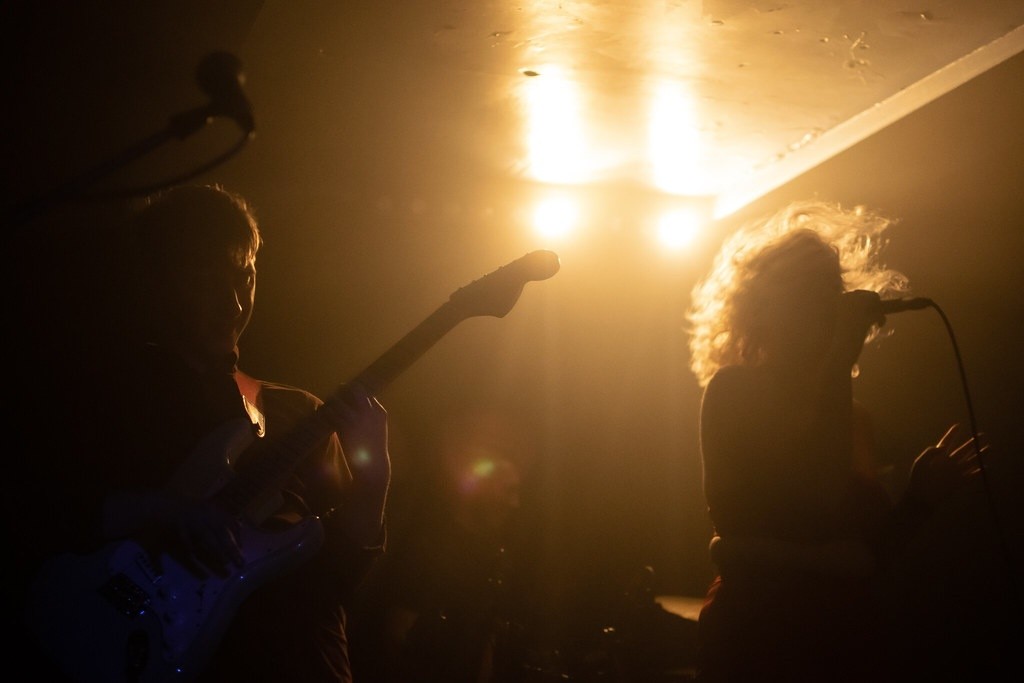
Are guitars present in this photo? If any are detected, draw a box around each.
[228,248,561,503]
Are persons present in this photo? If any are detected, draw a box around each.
[688,186,993,682]
[111,184,392,683]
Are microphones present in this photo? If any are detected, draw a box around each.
[880,295,934,314]
[197,50,257,133]
